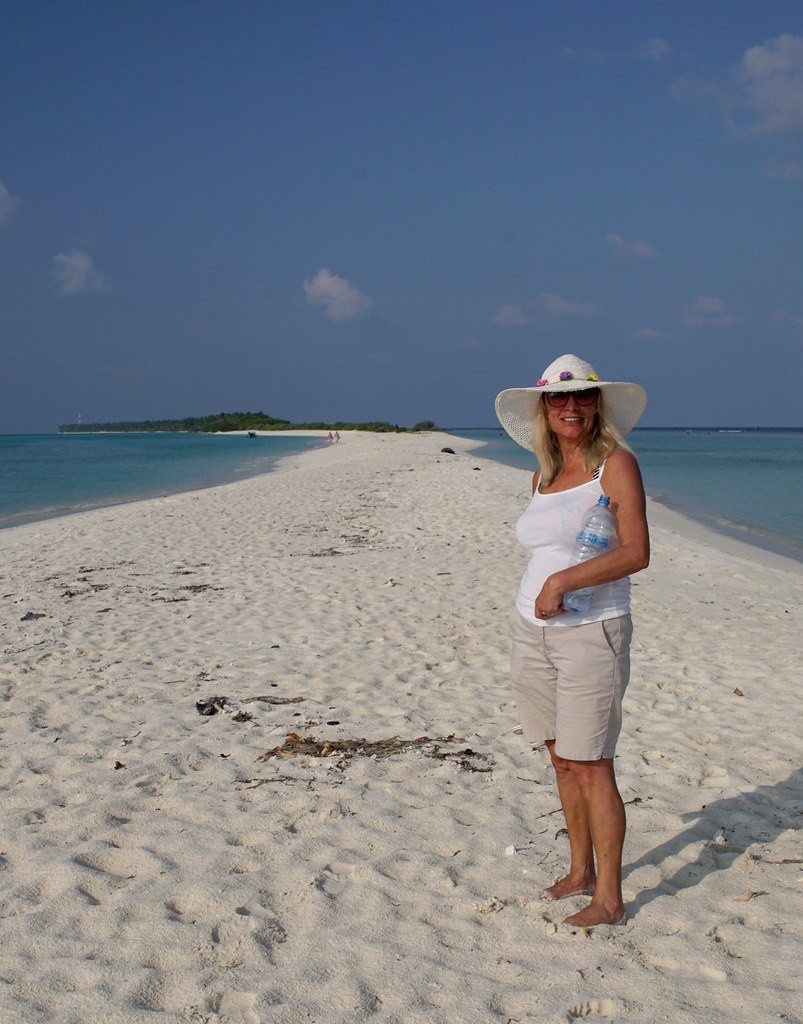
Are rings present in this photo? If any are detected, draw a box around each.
[551,613,554,616]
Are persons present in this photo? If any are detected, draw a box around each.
[494,354,651,928]
[328,431,333,444]
[335,431,339,443]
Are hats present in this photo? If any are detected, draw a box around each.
[495,354,648,453]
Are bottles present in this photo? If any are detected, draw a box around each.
[560,495,614,613]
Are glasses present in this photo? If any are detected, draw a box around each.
[546,388,595,407]
